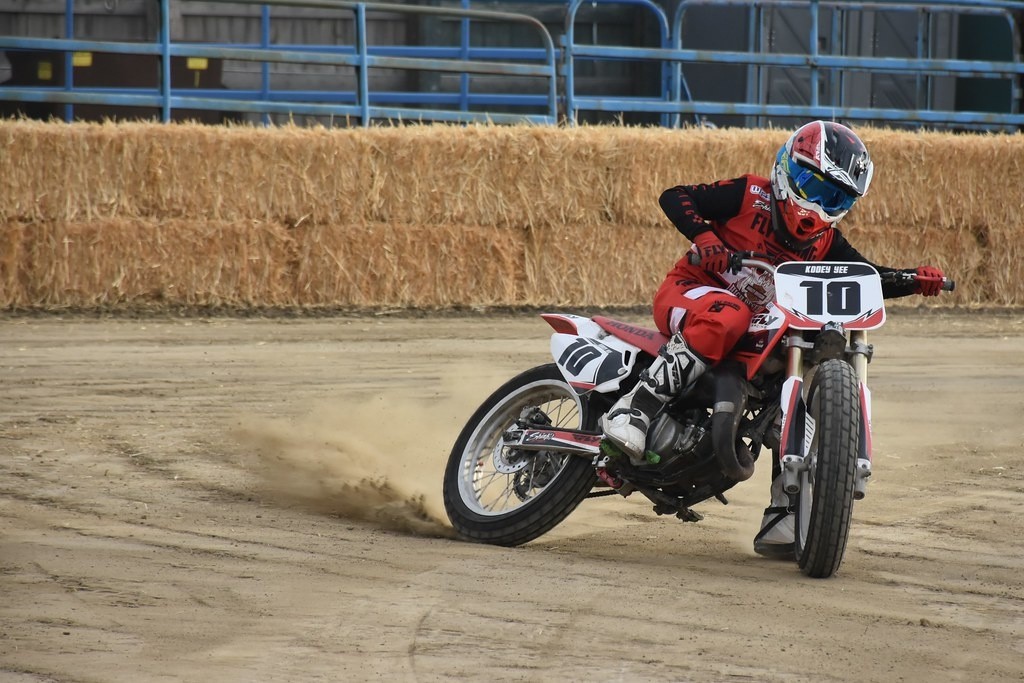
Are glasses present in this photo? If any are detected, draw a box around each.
[794,168,847,211]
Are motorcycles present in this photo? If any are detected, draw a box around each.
[442,247,955,579]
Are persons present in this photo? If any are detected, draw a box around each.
[598,120,944,560]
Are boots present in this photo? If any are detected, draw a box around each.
[754,424,798,561]
[598,331,714,462]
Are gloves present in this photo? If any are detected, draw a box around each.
[896,265,944,297]
[693,230,732,274]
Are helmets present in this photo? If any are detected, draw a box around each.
[770,121,874,252]
